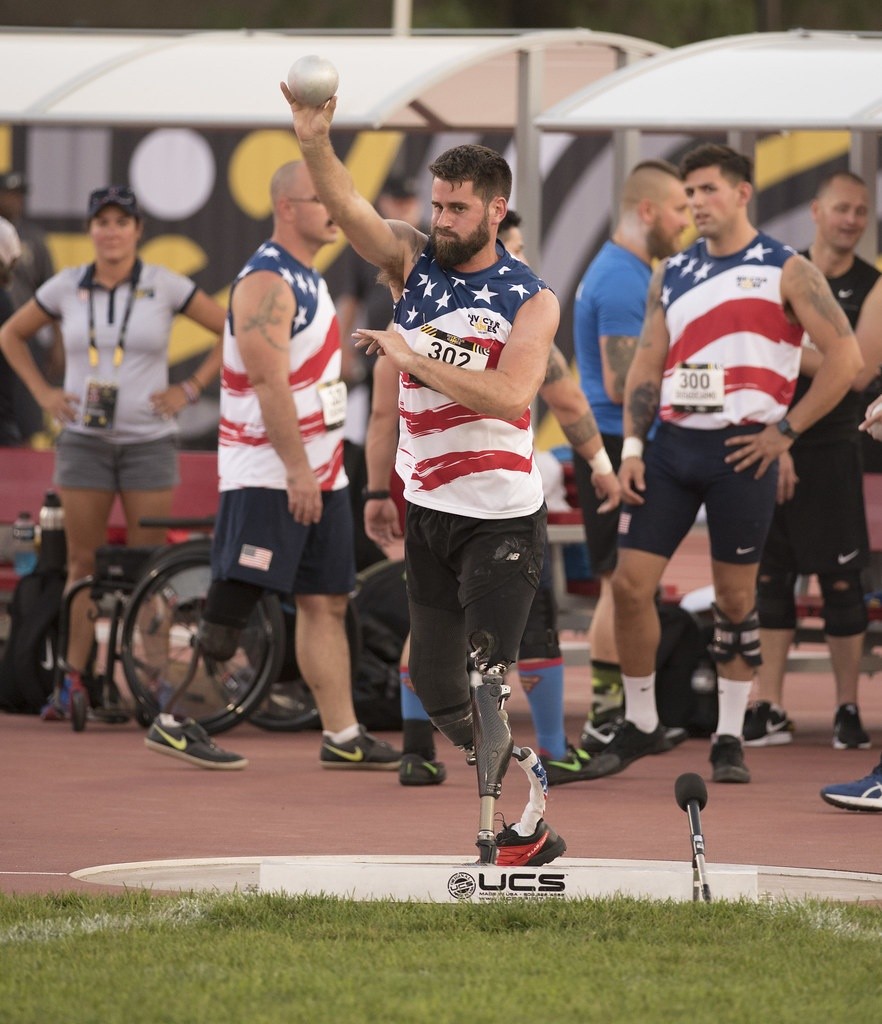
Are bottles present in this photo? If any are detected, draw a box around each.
[38,493,66,566]
[665,581,677,595]
[12,511,36,575]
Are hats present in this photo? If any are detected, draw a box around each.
[88,191,137,219]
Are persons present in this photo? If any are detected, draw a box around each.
[144,158,404,771]
[281,61,569,867]
[1,188,228,725]
[0,158,882,809]
[593,143,867,783]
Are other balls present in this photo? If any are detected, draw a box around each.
[287,54,340,107]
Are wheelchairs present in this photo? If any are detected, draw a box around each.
[53,514,322,734]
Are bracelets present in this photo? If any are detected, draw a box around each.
[187,373,205,396]
[359,486,390,499]
[620,437,644,460]
[588,447,614,475]
[178,380,199,405]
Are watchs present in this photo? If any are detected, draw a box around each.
[776,418,799,440]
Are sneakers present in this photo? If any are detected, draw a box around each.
[831,704,871,750]
[709,735,750,783]
[581,720,671,777]
[542,745,620,784]
[400,752,446,785]
[42,681,90,721]
[319,724,403,770]
[580,707,688,751]
[820,763,882,812]
[144,716,248,770]
[150,684,187,719]
[742,703,793,746]
[476,812,567,866]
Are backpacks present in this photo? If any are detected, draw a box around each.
[0,575,104,714]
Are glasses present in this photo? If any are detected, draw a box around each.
[91,187,133,205]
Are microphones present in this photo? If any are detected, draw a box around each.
[674,772,712,905]
